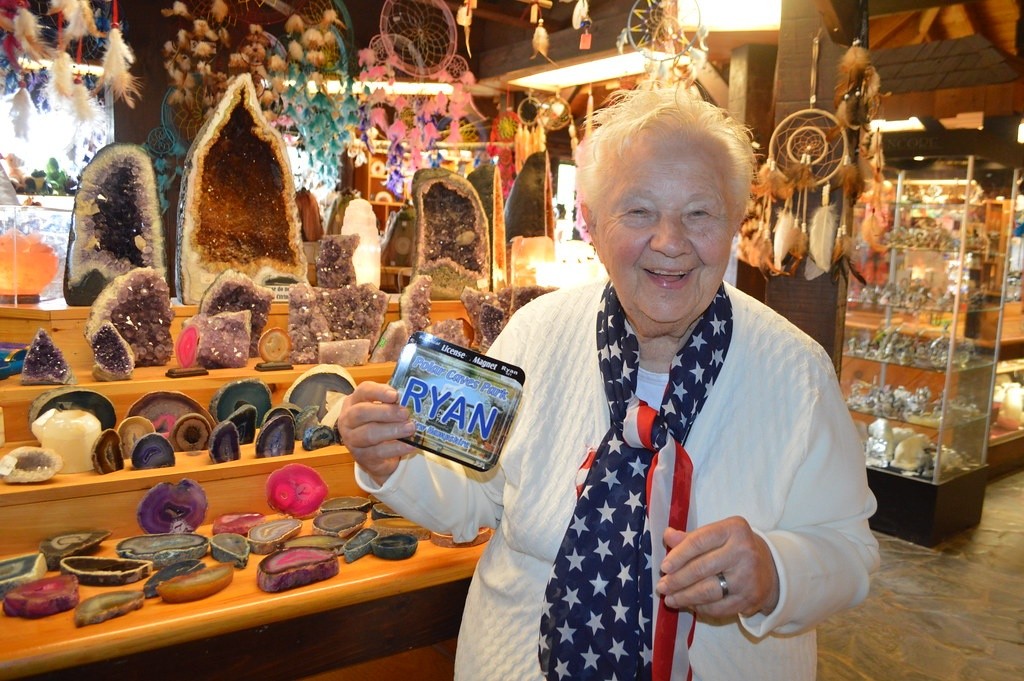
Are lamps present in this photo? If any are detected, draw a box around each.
[479,42,692,96]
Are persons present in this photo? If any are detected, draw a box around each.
[336,88,878,681]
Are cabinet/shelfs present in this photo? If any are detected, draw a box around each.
[844,128,1024,548]
[0,298,488,681]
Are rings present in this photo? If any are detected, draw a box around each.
[717,573,728,598]
